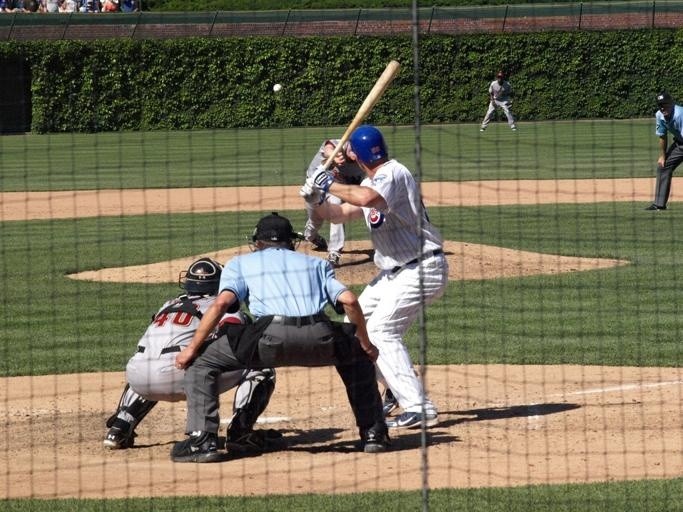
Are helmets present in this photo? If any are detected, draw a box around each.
[349,124,389,164]
[179,256,224,297]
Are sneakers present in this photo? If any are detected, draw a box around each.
[512,127,516,130]
[327,250,340,266]
[103,427,134,448]
[480,128,485,132]
[170,428,218,463]
[383,388,398,415]
[386,410,439,430]
[359,424,391,453]
[226,428,286,452]
[312,235,328,249]
[641,203,666,211]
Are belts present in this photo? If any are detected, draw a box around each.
[137,345,180,353]
[405,247,442,265]
[271,310,324,325]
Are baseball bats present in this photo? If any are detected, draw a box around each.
[322,59,400,167]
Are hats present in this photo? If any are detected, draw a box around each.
[252,212,305,242]
[654,93,672,106]
[496,71,504,78]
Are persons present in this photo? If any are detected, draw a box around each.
[102,257,281,450]
[480,73,518,132]
[171,213,393,463]
[299,125,449,430]
[300,139,351,266]
[1,0,140,13]
[644,93,682,211]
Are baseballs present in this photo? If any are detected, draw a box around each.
[272,84,283,93]
